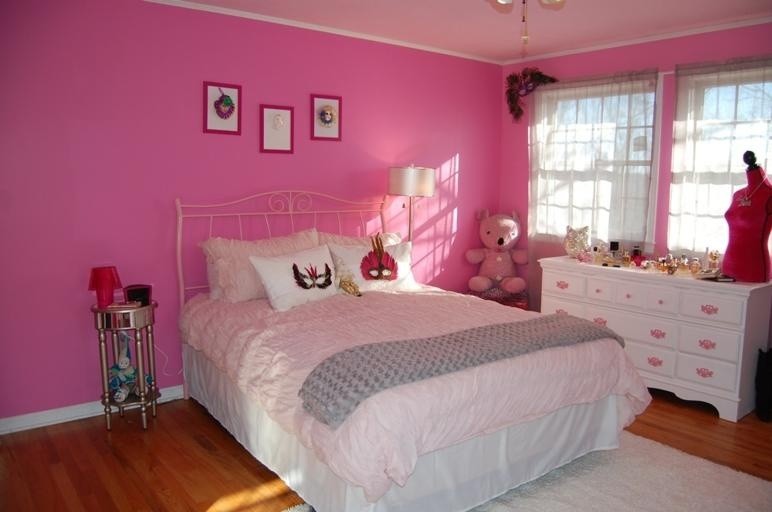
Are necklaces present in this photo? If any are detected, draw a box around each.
[737,175,769,209]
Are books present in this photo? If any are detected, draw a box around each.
[697,267,734,283]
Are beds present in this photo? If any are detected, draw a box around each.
[174,189,653,511]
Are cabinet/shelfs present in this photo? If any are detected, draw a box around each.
[536,256,772,424]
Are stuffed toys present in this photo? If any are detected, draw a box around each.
[463,206,529,296]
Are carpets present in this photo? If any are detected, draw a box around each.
[275,430,772,512]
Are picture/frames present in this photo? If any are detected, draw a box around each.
[202,80,242,136]
[310,94,343,142]
[259,103,295,154]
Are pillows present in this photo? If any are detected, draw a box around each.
[328,241,421,292]
[317,231,402,272]
[197,228,318,304]
[248,244,336,311]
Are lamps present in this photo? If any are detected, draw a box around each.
[487,0,566,59]
[388,163,434,240]
